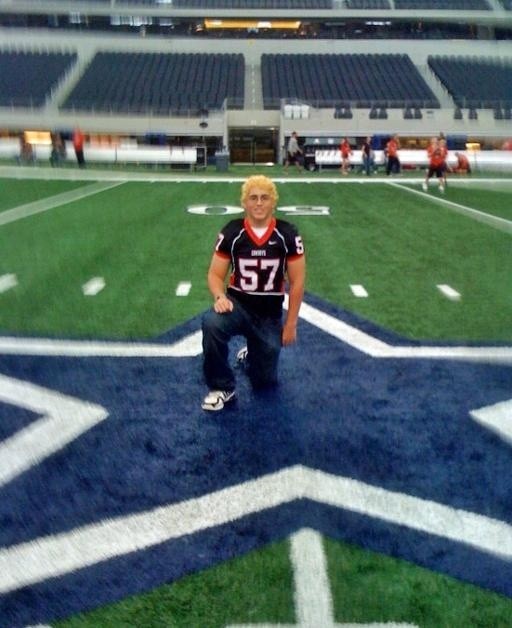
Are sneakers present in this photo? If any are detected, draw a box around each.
[201,390,235,413]
[236,345,249,367]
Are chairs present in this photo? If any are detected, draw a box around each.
[1,50,512,122]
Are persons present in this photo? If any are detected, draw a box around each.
[281,132,471,194]
[201,176,306,411]
[73,126,86,169]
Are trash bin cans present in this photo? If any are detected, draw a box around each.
[215,151,230,171]
